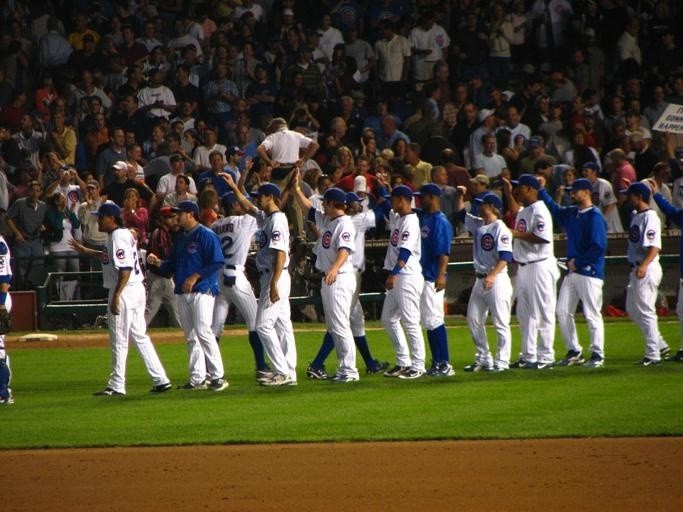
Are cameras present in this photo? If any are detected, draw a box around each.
[63,171,69,177]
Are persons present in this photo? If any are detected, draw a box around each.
[148,171,300,391]
[0,238,15,405]
[455,175,610,373]
[1,1,682,330]
[292,165,454,381]
[649,178,683,363]
[67,203,172,396]
[621,182,671,366]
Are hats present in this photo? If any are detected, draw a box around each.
[267,118,287,129]
[112,161,127,170]
[170,155,185,163]
[86,180,99,189]
[478,108,495,121]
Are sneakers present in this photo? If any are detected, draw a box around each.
[0,395,15,405]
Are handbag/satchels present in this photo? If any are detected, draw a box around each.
[271,167,293,180]
